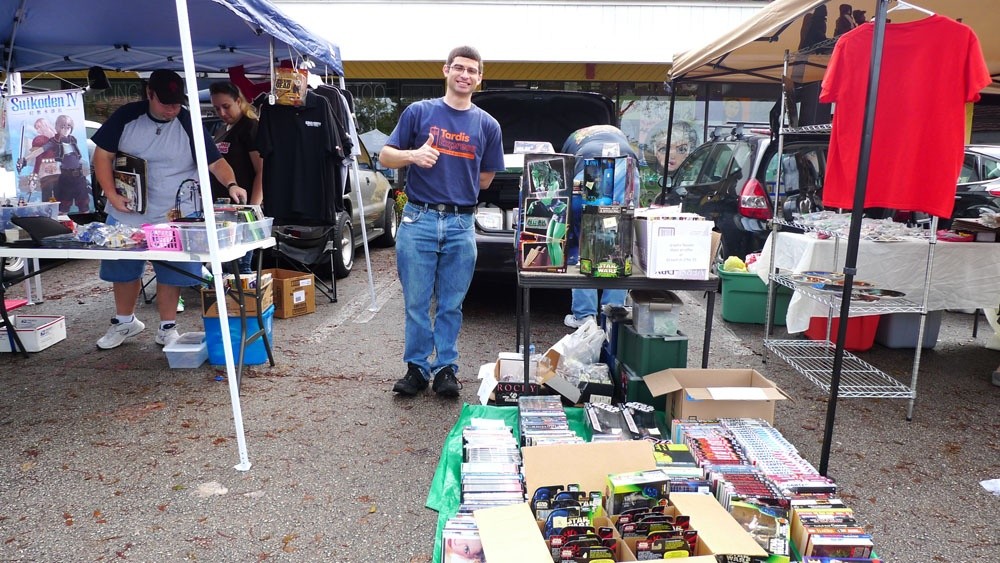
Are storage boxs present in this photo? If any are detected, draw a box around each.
[477,358,614,406]
[476,208,504,230]
[718,264,943,352]
[642,368,796,431]
[253,268,316,319]
[178,223,243,251]
[201,304,274,366]
[200,278,273,317]
[621,324,688,411]
[162,332,208,368]
[629,290,684,335]
[473,440,769,563]
[0,315,66,353]
[0,202,61,233]
[239,217,274,242]
[633,219,714,280]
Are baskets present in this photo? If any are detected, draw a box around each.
[140,222,193,251]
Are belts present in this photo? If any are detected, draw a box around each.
[408,197,477,213]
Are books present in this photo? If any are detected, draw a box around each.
[97,151,148,215]
[173,201,271,289]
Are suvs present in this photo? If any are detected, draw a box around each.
[654,121,911,293]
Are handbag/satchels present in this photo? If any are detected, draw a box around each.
[543,320,606,366]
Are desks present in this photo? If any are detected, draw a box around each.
[756,231,1000,338]
[0,238,277,396]
[516,255,719,395]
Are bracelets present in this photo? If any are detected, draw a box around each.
[227,183,237,190]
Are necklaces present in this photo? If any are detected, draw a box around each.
[150,112,164,135]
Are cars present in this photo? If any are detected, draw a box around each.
[911,144,1000,231]
[470,89,617,296]
[334,133,401,278]
[83,120,102,191]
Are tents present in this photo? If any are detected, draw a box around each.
[0,0,373,467]
[660,0,1000,476]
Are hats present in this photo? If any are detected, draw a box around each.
[149,69,188,106]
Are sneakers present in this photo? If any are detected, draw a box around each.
[155,323,180,345]
[431,366,463,397]
[392,360,429,397]
[177,296,185,311]
[97,314,145,349]
[564,314,591,327]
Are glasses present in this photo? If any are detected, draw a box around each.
[447,65,481,75]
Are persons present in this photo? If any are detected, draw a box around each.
[91,68,245,351]
[205,81,264,279]
[378,47,505,395]
[561,125,640,329]
[445,539,483,560]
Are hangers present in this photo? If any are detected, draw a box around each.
[300,60,324,90]
[871,0,934,21]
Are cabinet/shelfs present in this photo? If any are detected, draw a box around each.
[763,35,938,422]
